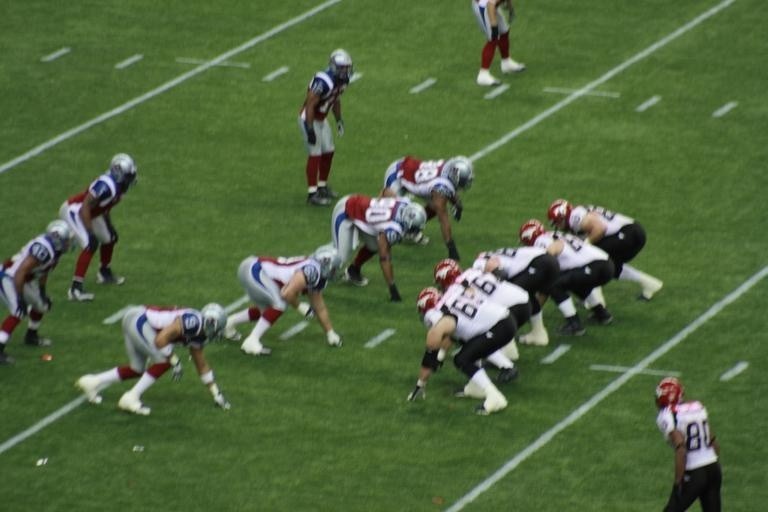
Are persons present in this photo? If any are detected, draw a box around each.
[330,194,428,302]
[471,1,527,88]
[0,219,72,365]
[58,153,137,301]
[406,200,665,416]
[297,48,355,207]
[652,376,724,512]
[74,302,230,416]
[377,154,475,262]
[221,245,343,356]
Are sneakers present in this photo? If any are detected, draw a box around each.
[25,338,51,346]
[241,341,271,357]
[561,303,611,330]
[74,376,150,415]
[638,274,662,297]
[223,326,242,341]
[477,69,499,86]
[0,355,14,365]
[501,59,525,74]
[459,367,517,413]
[405,231,430,246]
[308,187,339,202]
[70,268,126,299]
[344,268,369,287]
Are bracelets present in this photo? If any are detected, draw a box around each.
[199,369,215,386]
[160,343,175,358]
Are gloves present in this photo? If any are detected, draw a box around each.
[212,392,230,411]
[388,284,401,302]
[89,227,118,248]
[450,194,462,221]
[336,120,343,136]
[326,331,343,348]
[16,292,27,317]
[446,240,460,258]
[39,285,51,310]
[408,379,427,402]
[307,126,315,144]
[297,303,315,320]
[170,360,182,384]
[491,25,498,38]
[672,483,683,498]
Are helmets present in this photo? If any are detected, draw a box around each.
[111,154,137,184]
[520,197,572,241]
[449,160,473,189]
[329,49,353,80]
[419,261,461,310]
[200,303,227,344]
[314,247,341,278]
[655,377,683,408]
[46,220,76,253]
[402,203,427,230]
